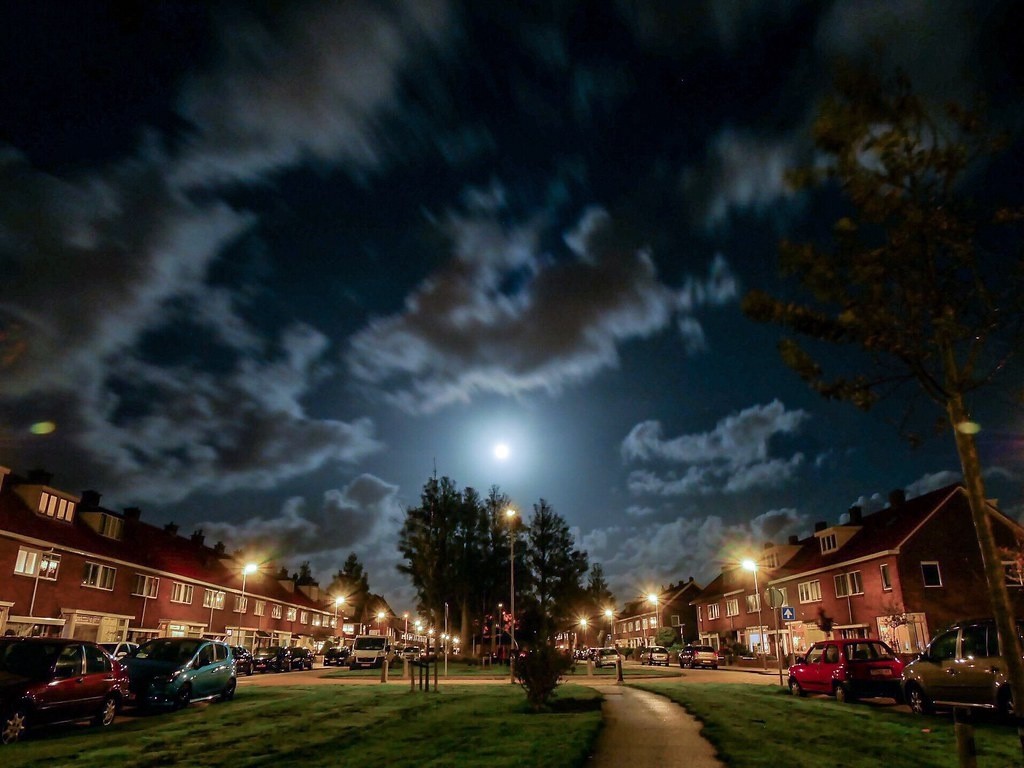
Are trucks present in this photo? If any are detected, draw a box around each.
[353,634,395,669]
[396,641,461,664]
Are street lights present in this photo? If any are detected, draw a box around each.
[403,613,409,646]
[333,595,344,631]
[649,593,660,646]
[377,612,384,629]
[504,507,517,683]
[236,561,258,644]
[580,618,588,645]
[747,562,768,670]
[607,610,614,646]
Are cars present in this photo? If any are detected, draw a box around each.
[593,647,622,668]
[900,618,1019,727]
[571,647,597,661]
[230,645,254,676]
[96,641,147,661]
[288,646,306,672]
[118,637,237,715]
[252,646,292,674]
[640,646,670,668]
[678,644,719,670]
[787,638,907,704]
[303,648,314,670]
[324,646,353,666]
[0,635,130,747]
